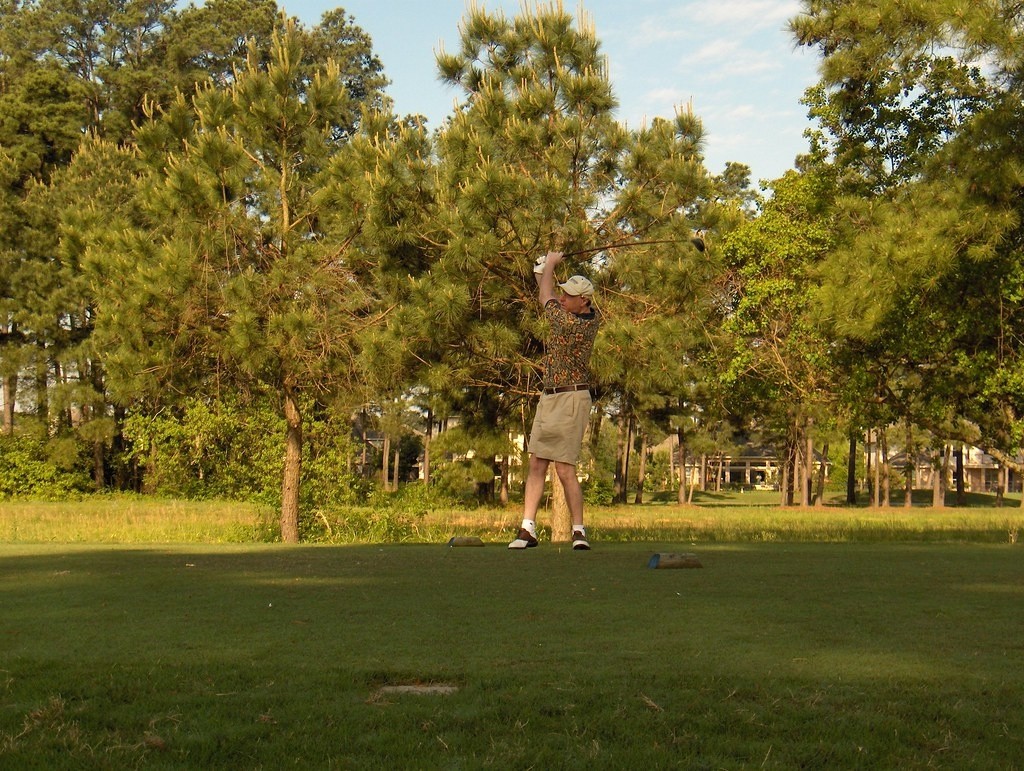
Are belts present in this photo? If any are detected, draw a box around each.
[544,384,589,395]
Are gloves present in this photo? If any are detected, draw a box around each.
[534,256,546,273]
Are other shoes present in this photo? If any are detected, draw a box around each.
[508,528,538,549]
[572,530,590,550]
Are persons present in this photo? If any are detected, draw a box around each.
[508,251,597,548]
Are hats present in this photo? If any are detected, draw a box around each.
[555,275,594,300]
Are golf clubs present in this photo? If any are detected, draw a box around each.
[534,238,706,266]
